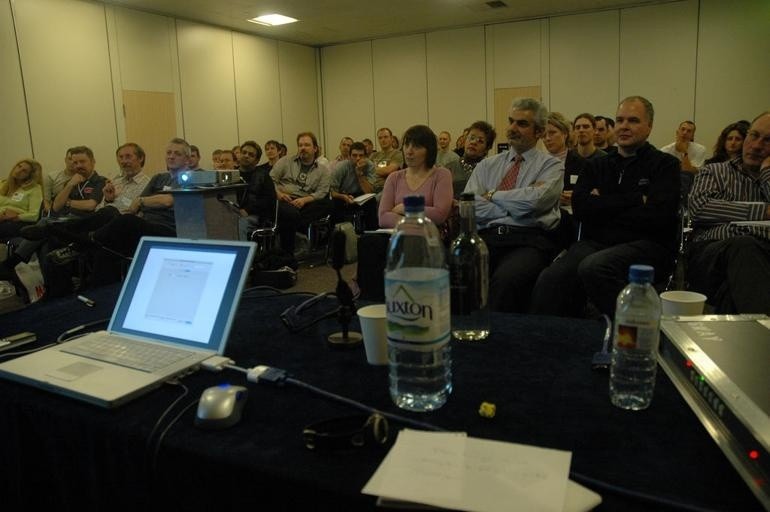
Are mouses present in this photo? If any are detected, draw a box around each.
[194,383,249,426]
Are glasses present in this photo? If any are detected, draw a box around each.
[466,135,486,144]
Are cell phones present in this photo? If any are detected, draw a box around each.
[0,331,37,352]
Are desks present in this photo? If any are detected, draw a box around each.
[0,283,770,512]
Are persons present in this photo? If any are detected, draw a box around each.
[1,112,770,325]
[451,97,567,318]
[529,96,684,317]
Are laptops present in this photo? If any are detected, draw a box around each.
[0,234,257,412]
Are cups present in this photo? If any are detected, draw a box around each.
[658,287,707,315]
[356,304,390,367]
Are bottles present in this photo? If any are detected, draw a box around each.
[454,191,493,344]
[608,265,661,413]
[383,194,454,412]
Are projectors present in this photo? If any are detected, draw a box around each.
[177,169,241,186]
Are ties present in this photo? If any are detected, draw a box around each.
[497,155,524,191]
